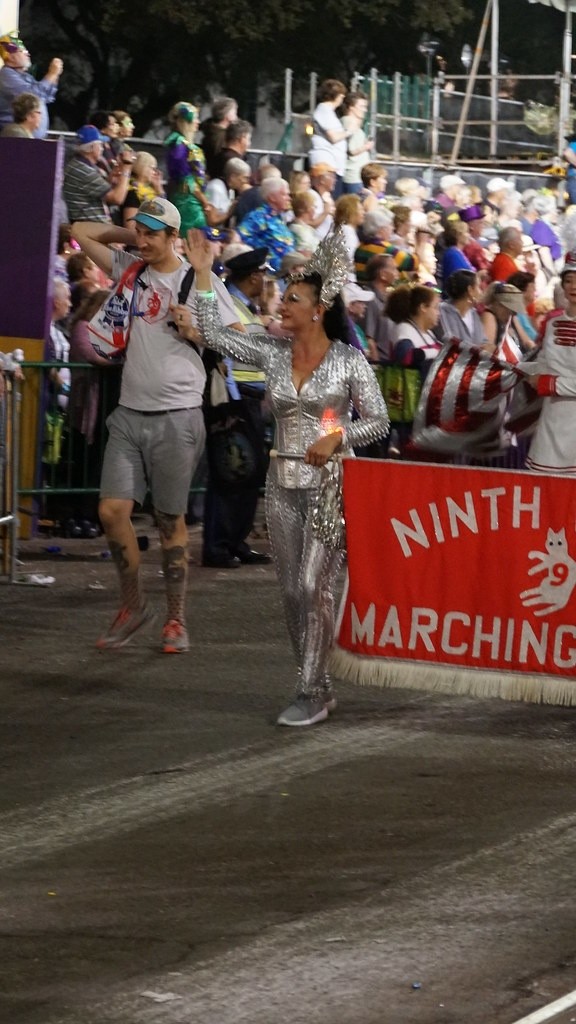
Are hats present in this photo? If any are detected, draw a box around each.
[458,205,486,221]
[223,246,277,276]
[494,283,524,312]
[486,177,513,193]
[77,124,111,144]
[310,162,337,176]
[440,174,466,188]
[560,249,576,274]
[340,283,375,305]
[520,235,540,252]
[362,205,395,234]
[125,197,182,234]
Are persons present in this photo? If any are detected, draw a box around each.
[0,30,576,571]
[72,196,246,652]
[184,228,390,728]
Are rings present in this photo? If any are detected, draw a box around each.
[180,315,182,320]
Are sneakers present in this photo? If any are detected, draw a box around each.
[323,693,335,711]
[98,600,153,649]
[161,617,188,653]
[275,690,329,726]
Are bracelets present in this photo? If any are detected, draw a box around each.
[197,290,216,297]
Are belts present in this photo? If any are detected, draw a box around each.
[128,407,193,417]
[234,384,265,401]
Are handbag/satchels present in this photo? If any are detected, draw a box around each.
[373,363,420,423]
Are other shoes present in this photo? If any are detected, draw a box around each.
[238,551,273,566]
[201,549,239,568]
